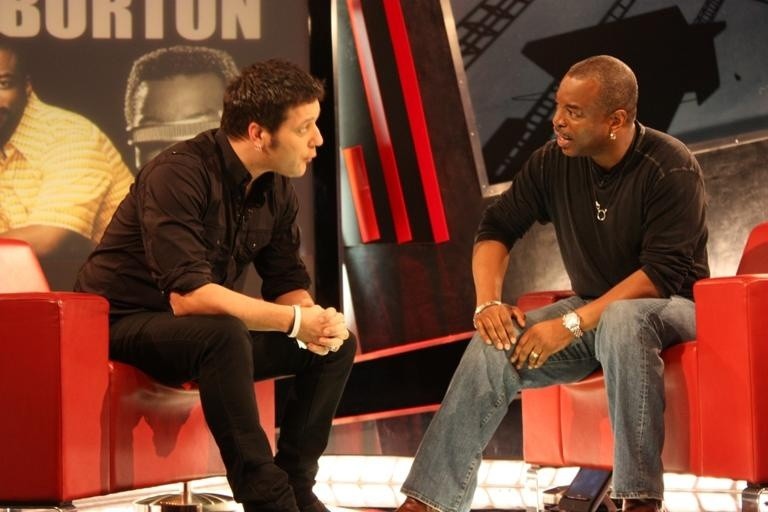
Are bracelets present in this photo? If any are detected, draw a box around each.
[288,304,302,338]
[472,300,502,330]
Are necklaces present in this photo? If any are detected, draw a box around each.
[586,145,627,223]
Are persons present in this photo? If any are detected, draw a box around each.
[392,51,715,511]
[80,56,359,512]
[122,45,247,171]
[0,31,139,298]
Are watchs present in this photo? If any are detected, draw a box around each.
[562,311,585,337]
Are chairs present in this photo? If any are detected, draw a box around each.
[514,220,768,511]
[0,234,277,509]
